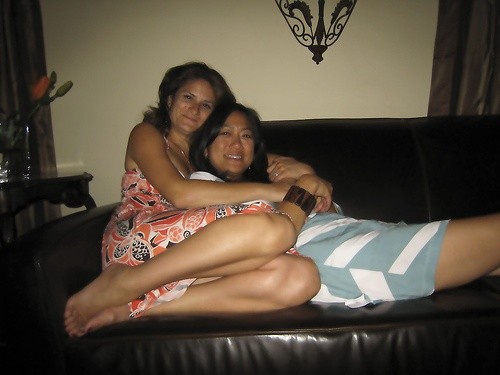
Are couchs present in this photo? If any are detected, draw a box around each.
[13,114,500,375]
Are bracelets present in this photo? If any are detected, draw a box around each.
[283,184,316,218]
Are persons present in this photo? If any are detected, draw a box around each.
[63,61,321,341]
[190,102,500,308]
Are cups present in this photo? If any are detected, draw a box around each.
[0,153,14,184]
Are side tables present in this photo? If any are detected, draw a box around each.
[0,171,96,245]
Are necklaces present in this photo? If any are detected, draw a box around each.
[174,144,185,157]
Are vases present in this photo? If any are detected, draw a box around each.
[3,113,38,176]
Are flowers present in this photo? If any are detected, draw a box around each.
[0,71,73,152]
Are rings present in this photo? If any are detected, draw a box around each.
[275,171,279,177]
